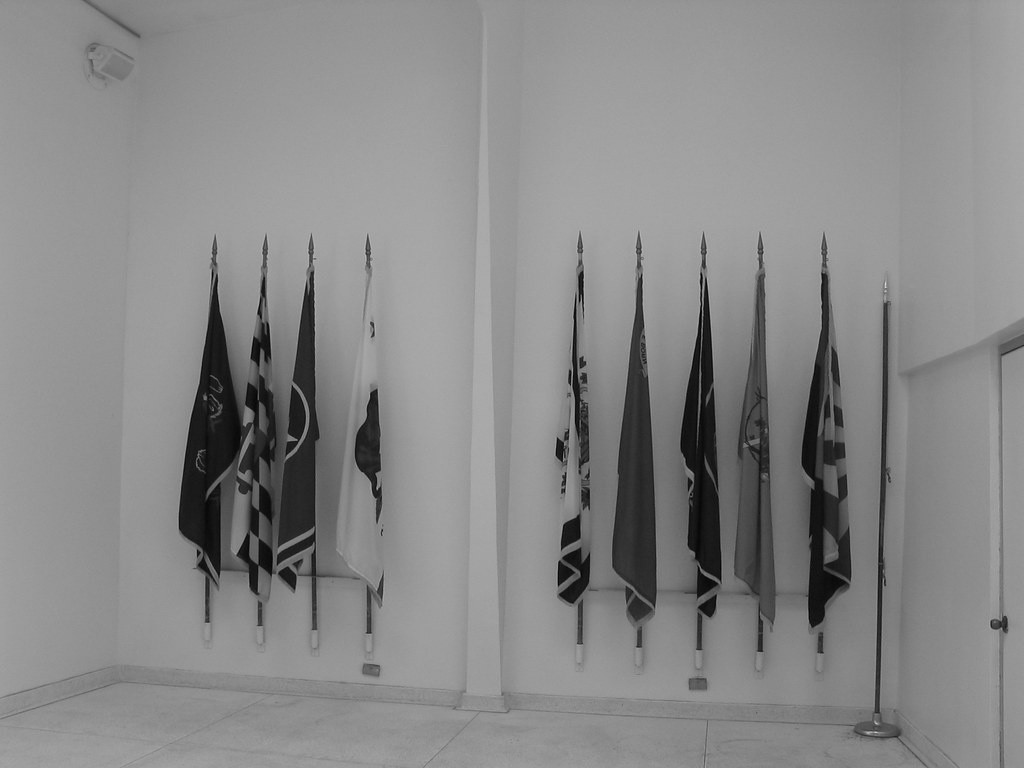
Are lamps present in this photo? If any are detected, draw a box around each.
[84,42,134,86]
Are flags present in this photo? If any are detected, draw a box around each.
[177,271,240,591]
[735,271,776,633]
[801,295,853,649]
[231,274,275,603]
[678,269,723,619]
[611,279,658,629]
[277,269,319,593]
[553,261,592,607]
[333,275,384,609]
